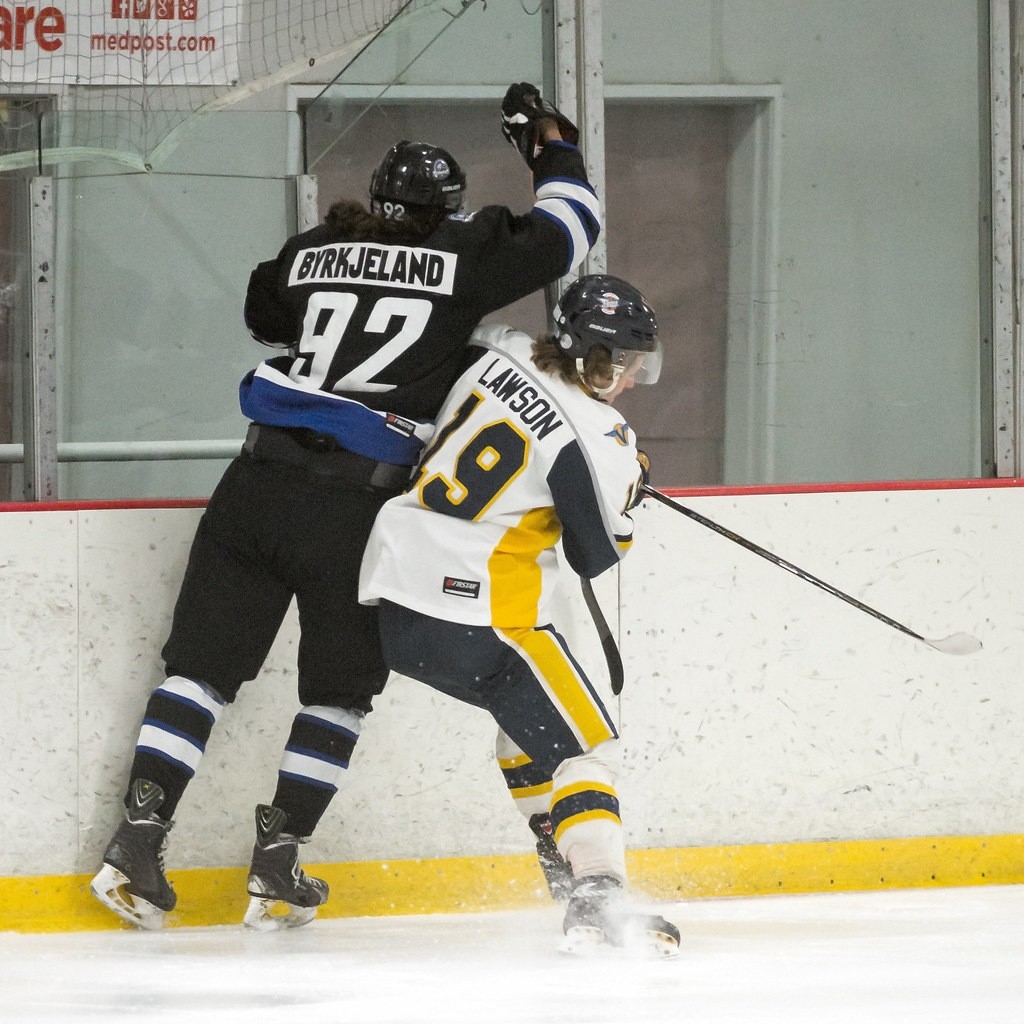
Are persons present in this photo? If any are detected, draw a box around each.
[354,272,685,961]
[93,81,603,928]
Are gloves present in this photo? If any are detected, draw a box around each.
[500,82,580,172]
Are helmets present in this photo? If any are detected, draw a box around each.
[551,274,660,394]
[368,140,467,213]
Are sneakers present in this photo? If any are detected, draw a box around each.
[558,876,680,965]
[89,778,177,930]
[242,804,329,933]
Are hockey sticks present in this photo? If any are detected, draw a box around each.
[542,283,984,696]
